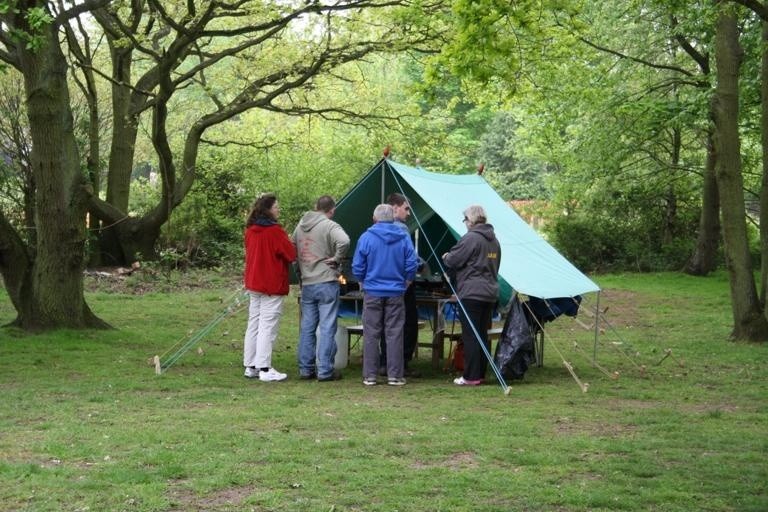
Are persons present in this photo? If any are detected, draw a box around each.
[442,206,501,386]
[380,192,421,377]
[290,196,350,381]
[352,204,418,385]
[244,195,296,382]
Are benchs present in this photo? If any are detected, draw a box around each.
[483,327,505,357]
[346,321,427,366]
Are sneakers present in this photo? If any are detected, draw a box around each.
[243,366,260,378]
[362,377,377,385]
[300,371,318,381]
[403,368,424,379]
[387,377,408,386]
[378,367,387,376]
[319,370,343,381]
[453,376,487,387]
[259,367,288,382]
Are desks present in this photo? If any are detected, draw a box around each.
[297,294,459,370]
